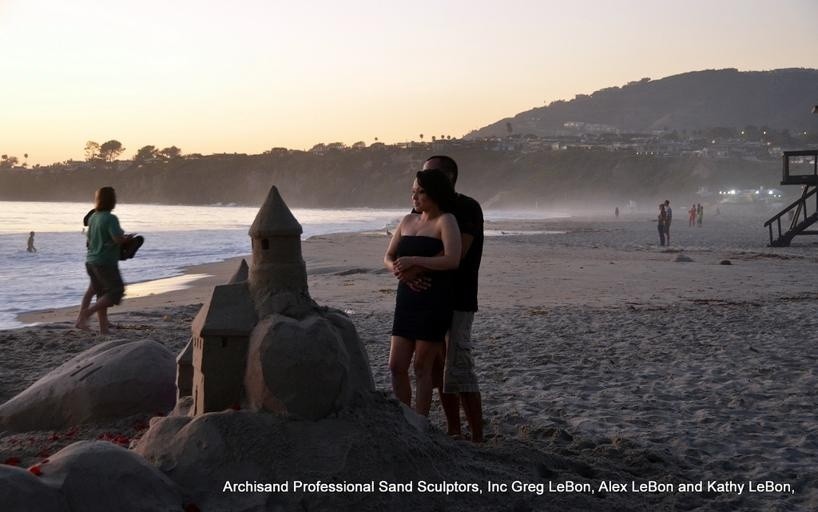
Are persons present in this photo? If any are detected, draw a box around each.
[25,231,37,253]
[651,204,667,246]
[77,187,137,337]
[696,203,701,224]
[662,200,673,245]
[688,205,697,227]
[393,155,484,443]
[74,208,118,331]
[383,168,463,418]
[699,206,704,225]
